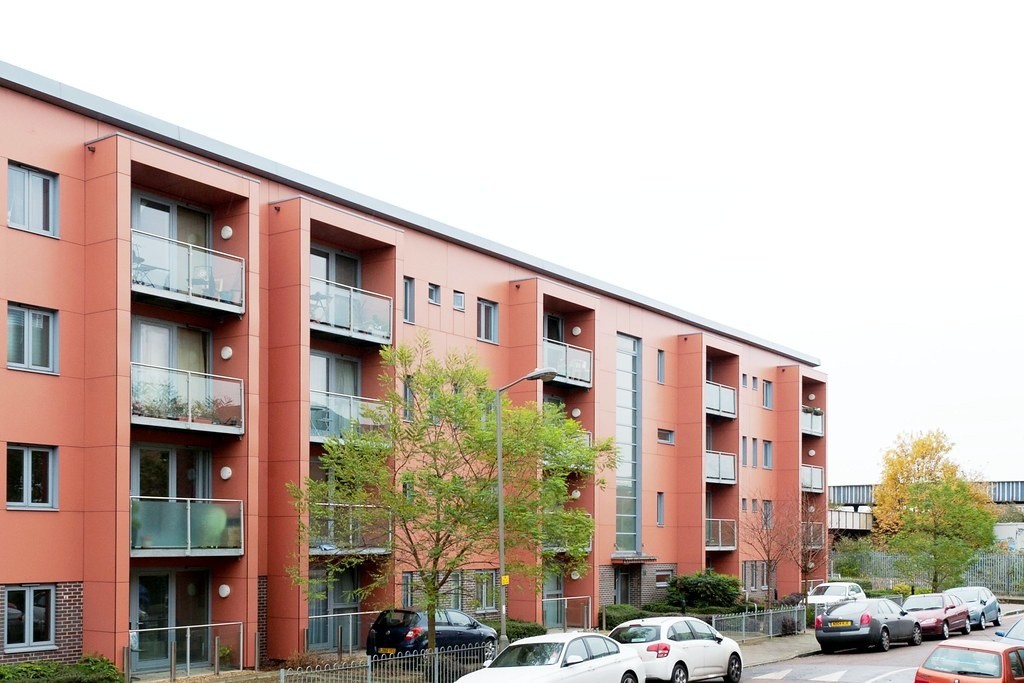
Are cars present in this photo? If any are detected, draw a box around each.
[945,585,1001,629]
[915,636,1024,683]
[606,615,742,683]
[453,632,646,683]
[797,582,865,610]
[365,605,498,661]
[993,617,1024,643]
[815,598,922,652]
[901,592,971,639]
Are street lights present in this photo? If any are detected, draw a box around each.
[493,366,559,659]
